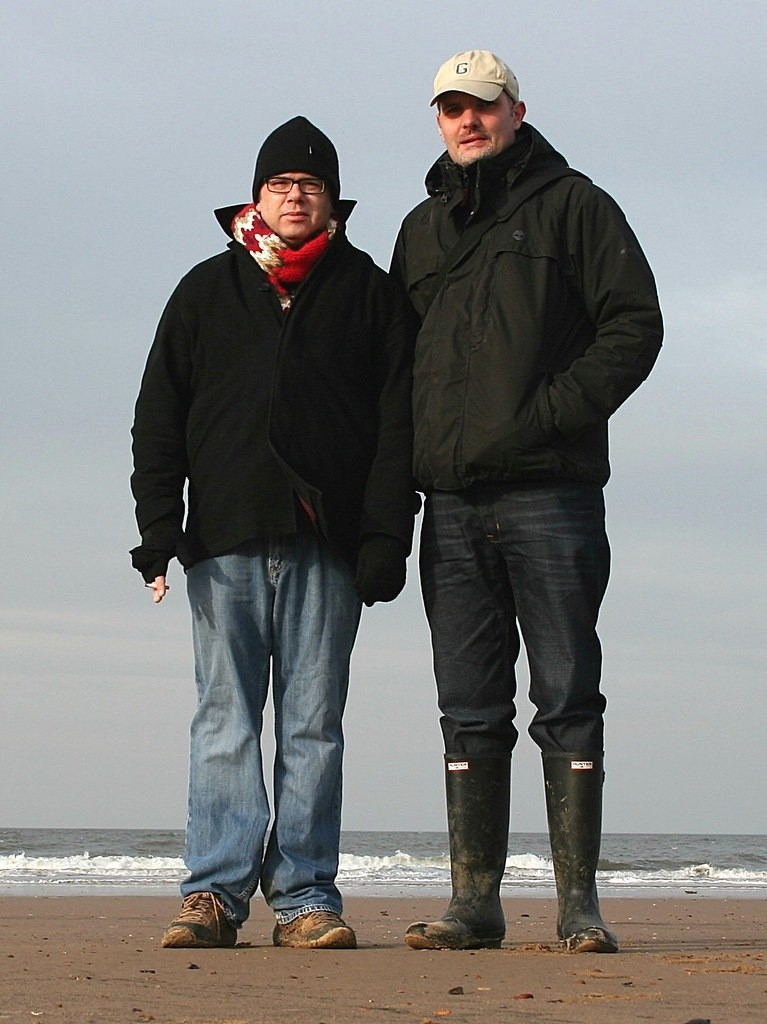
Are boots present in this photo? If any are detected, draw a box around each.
[541,749,617,952]
[404,751,512,950]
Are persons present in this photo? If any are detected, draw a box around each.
[129,114,423,948]
[388,47,665,954]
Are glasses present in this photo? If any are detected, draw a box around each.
[262,175,328,194]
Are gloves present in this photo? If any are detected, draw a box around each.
[353,533,408,607]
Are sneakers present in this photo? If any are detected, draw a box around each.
[273,905,357,949]
[161,883,237,950]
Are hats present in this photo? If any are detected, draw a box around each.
[252,116,341,204]
[429,49,519,107]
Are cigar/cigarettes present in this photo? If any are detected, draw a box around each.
[144,583,169,590]
